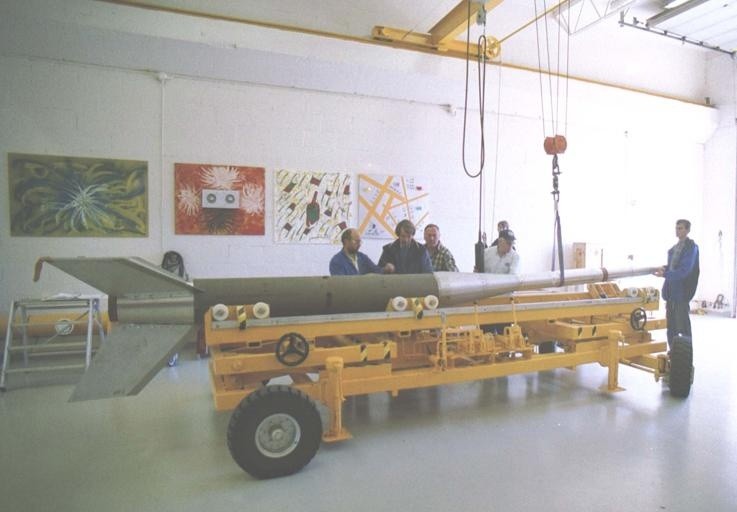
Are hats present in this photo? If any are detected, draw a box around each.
[499,230,514,240]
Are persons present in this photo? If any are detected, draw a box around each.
[422,223,458,273]
[330,229,394,276]
[652,220,699,399]
[482,221,515,251]
[472,230,518,275]
[377,219,433,274]
